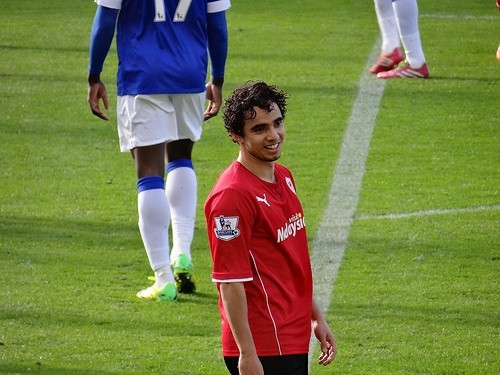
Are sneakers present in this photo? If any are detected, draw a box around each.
[174,255,198,293]
[370,47,404,74]
[136,284,179,302]
[377,64,429,78]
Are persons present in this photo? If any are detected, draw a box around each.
[369,0,430,79]
[86,0,232,301]
[203,78,338,375]
